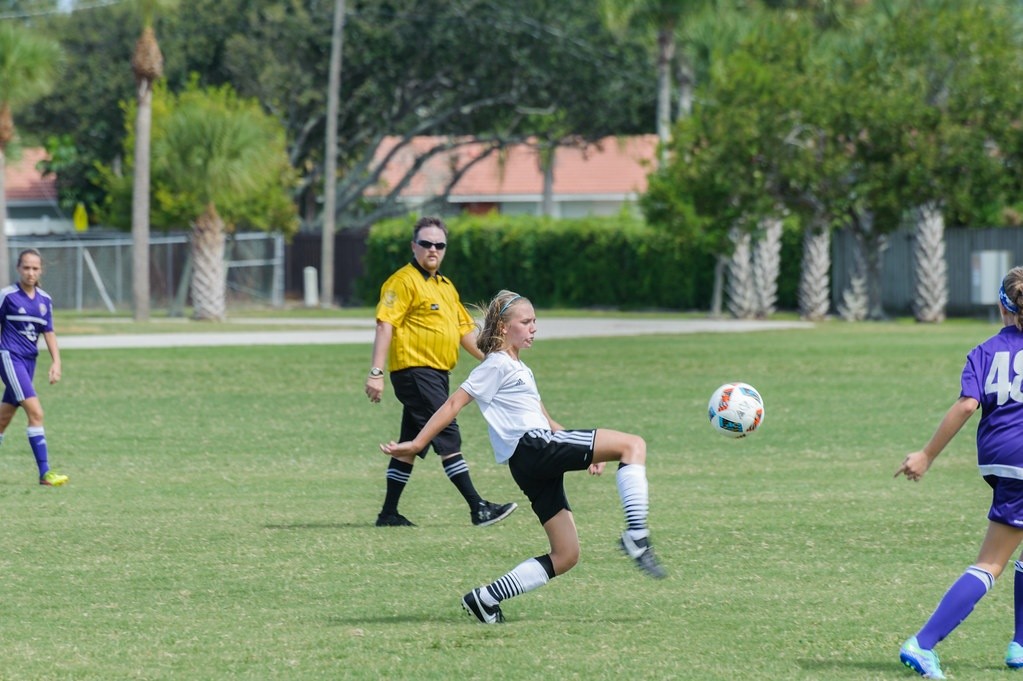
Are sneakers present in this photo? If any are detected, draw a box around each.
[39,470,68,486]
[1005,642,1023,669]
[470,500,518,527]
[898,636,948,679]
[461,588,506,624]
[617,530,667,580]
[376,513,417,527]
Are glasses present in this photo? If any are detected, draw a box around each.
[415,240,447,250]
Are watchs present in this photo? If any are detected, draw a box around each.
[369,367,384,378]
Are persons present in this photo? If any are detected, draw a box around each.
[379,290,672,624]
[893,265,1023,679]
[0,247,69,486]
[365,218,517,526]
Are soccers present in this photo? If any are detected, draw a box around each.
[707,381,765,439]
